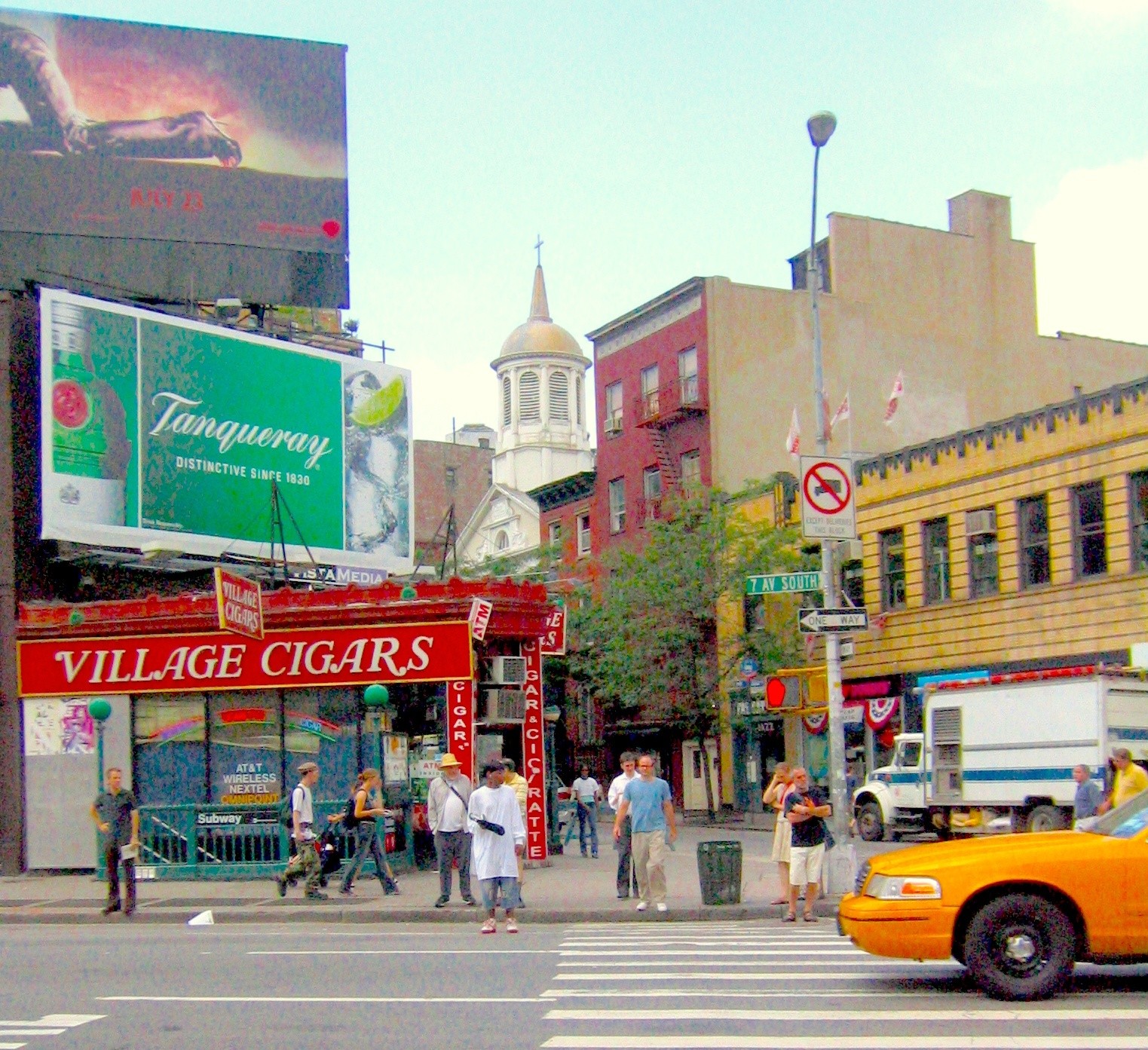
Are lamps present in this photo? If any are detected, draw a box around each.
[215,298,242,320]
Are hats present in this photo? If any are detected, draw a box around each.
[298,762,317,773]
[440,753,462,768]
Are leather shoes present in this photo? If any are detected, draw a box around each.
[617,893,629,899]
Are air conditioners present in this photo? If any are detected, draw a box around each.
[836,539,863,561]
[604,418,622,434]
[481,654,526,686]
[478,689,526,724]
[965,510,998,536]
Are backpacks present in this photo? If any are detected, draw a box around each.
[343,789,367,830]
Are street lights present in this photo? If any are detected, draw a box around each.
[802,107,858,843]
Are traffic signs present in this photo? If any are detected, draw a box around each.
[797,606,869,634]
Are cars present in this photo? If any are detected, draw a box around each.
[832,785,1148,1002]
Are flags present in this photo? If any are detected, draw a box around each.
[786,406,805,463]
[883,369,905,426]
[830,390,850,435]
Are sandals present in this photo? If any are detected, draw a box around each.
[803,911,818,921]
[781,911,795,922]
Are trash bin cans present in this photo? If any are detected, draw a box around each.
[697,841,743,905]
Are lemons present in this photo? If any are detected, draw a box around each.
[348,375,404,426]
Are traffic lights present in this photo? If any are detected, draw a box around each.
[763,674,803,712]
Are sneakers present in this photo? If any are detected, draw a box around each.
[481,918,498,933]
[461,892,476,907]
[636,902,648,912]
[434,895,451,908]
[656,902,667,913]
[505,917,518,933]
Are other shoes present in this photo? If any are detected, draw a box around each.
[123,898,136,915]
[102,900,121,914]
[771,896,789,905]
[382,883,400,895]
[272,873,287,897]
[337,886,357,898]
[304,888,328,901]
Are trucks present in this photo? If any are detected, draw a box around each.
[848,658,1148,843]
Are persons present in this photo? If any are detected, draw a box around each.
[89,768,139,915]
[327,768,403,897]
[608,752,679,913]
[780,765,833,922]
[1096,747,1148,817]
[427,753,476,908]
[762,761,807,906]
[1072,764,1106,831]
[572,765,600,860]
[275,761,329,902]
[468,758,529,933]
[844,763,859,807]
